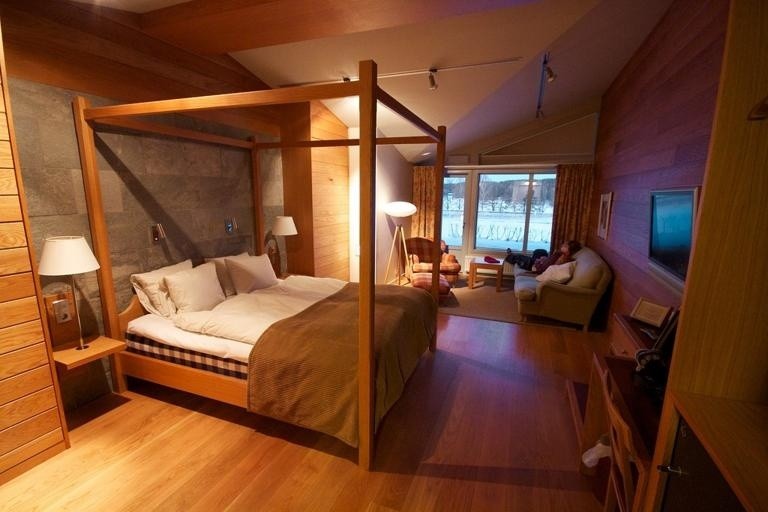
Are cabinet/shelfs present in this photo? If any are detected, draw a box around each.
[51,336,127,371]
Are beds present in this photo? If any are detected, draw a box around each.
[71,58,450,470]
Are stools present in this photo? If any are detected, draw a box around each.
[410,272,451,305]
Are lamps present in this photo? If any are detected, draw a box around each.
[149,223,167,243]
[426,73,439,90]
[272,216,299,276]
[542,59,546,83]
[37,235,100,350]
[383,201,417,286]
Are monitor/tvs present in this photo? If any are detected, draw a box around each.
[646,185,699,293]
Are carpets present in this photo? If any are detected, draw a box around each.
[402,270,577,331]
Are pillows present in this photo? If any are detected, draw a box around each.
[224,253,278,295]
[134,260,194,319]
[535,262,568,281]
[203,252,250,297]
[163,262,225,314]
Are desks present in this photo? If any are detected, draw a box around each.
[469,255,505,292]
[568,309,671,509]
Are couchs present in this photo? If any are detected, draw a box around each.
[408,238,461,287]
[515,248,611,332]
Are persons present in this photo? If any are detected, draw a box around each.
[506,240,581,275]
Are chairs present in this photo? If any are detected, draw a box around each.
[597,372,636,512]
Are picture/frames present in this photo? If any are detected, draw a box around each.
[596,192,613,241]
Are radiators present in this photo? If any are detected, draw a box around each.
[464,255,515,275]
